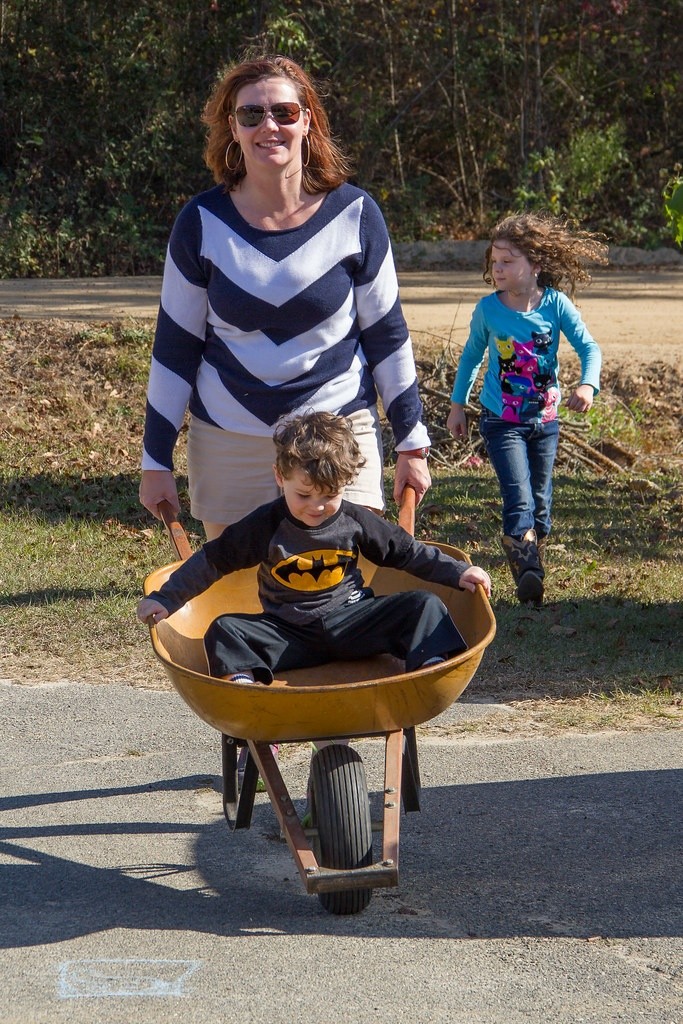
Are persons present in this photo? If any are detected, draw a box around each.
[447,207,610,608]
[137,407,491,685]
[139,55,432,829]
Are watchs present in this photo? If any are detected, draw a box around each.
[397,447,430,458]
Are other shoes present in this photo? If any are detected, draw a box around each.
[236,742,279,792]
[299,777,319,838]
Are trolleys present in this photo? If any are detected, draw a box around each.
[139,501,498,918]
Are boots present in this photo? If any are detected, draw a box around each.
[500,528,550,605]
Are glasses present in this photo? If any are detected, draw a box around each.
[229,101,306,127]
